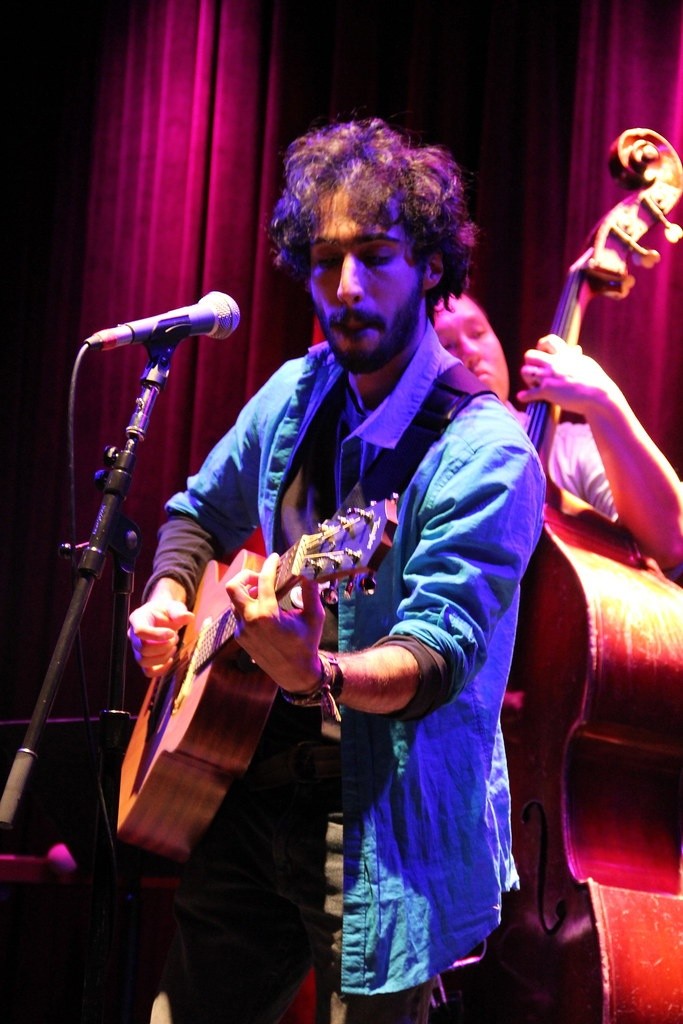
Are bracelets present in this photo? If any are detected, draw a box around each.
[281,649,344,722]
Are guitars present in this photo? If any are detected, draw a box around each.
[116,491,401,864]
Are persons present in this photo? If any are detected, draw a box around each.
[128,116,546,1024]
[435,291,683,572]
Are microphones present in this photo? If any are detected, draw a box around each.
[86,291,240,352]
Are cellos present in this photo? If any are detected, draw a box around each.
[453,122,683,1024]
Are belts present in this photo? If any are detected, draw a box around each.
[242,741,342,793]
